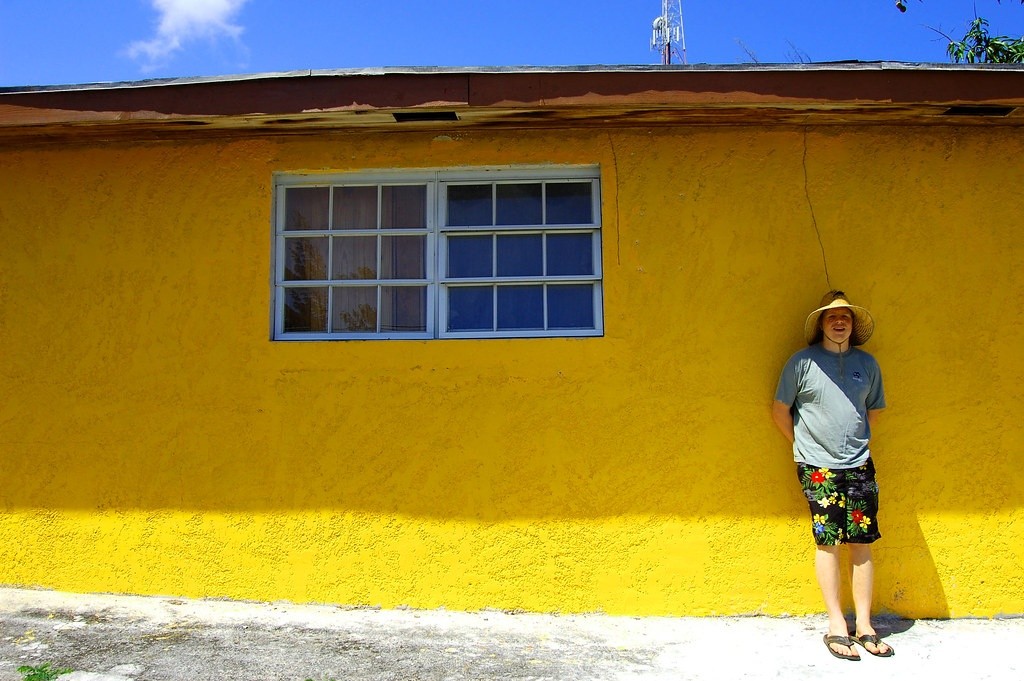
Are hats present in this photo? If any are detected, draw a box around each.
[804,291,874,347]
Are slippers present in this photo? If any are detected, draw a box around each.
[849,630,892,657]
[824,634,861,660]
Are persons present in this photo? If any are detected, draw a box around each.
[772,291,895,660]
